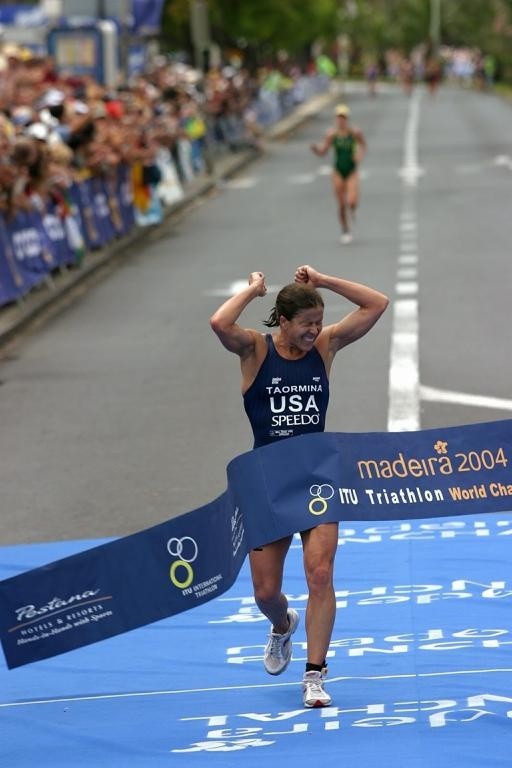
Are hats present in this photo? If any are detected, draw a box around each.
[336,104,349,118]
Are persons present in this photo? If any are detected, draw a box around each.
[208,263,390,709]
[364,43,497,103]
[1,39,332,221]
[309,103,371,243]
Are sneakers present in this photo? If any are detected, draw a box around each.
[264,608,300,676]
[302,669,332,708]
